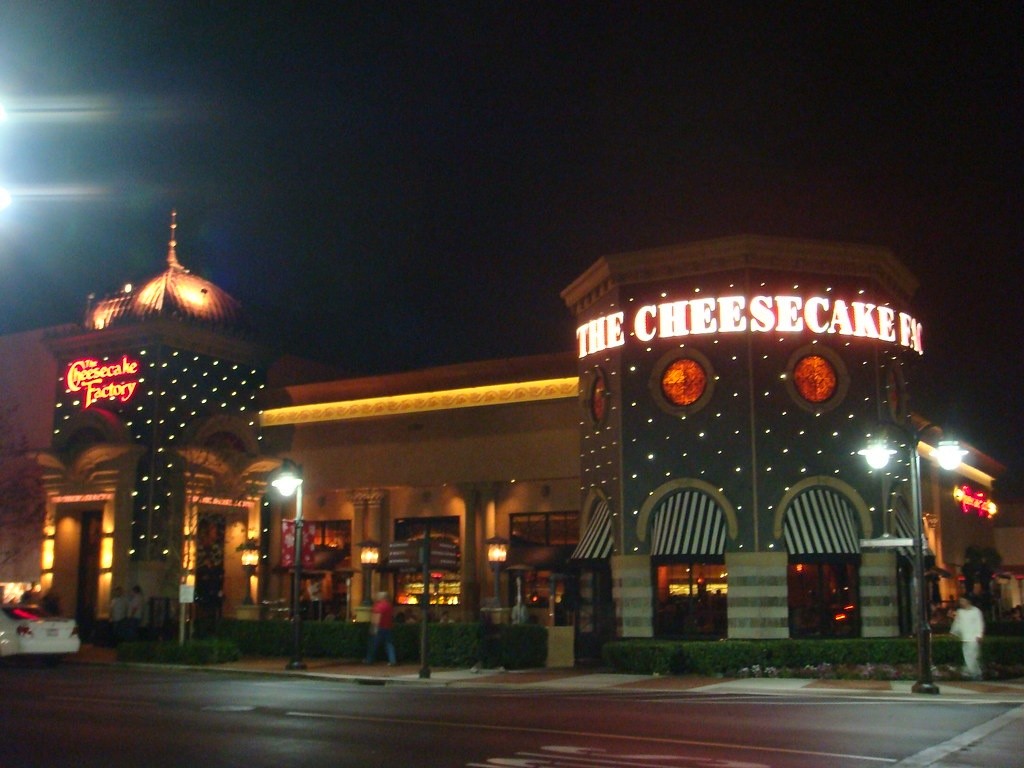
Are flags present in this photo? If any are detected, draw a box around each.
[279,518,316,572]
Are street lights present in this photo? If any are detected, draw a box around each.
[270,457,309,670]
[858,417,970,695]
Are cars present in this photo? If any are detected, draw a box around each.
[0,601,82,668]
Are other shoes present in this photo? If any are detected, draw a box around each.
[385,662,393,667]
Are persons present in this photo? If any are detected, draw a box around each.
[325,590,530,667]
[22,581,144,644]
[950,592,984,679]
